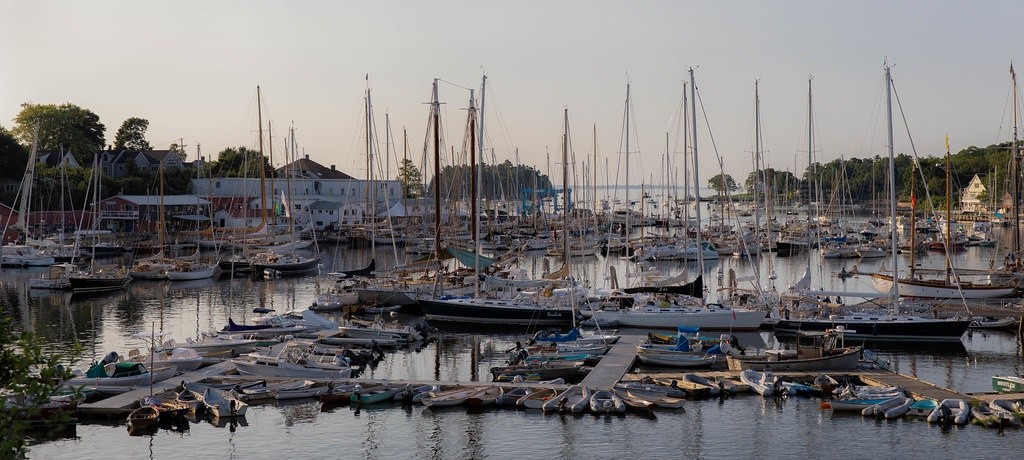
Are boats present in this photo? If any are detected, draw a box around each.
[712,375,750,393]
[682,373,721,395]
[2,308,437,432]
[992,375,1024,393]
[420,386,487,408]
[614,389,686,412]
[589,388,626,414]
[621,376,711,400]
[971,403,1016,429]
[634,319,866,370]
[740,368,791,397]
[543,386,592,413]
[524,386,557,408]
[488,344,608,384]
[466,384,503,408]
[796,310,968,427]
[989,398,1024,427]
[496,385,532,406]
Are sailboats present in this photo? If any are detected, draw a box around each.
[0,84,322,297]
[315,56,1024,344]
